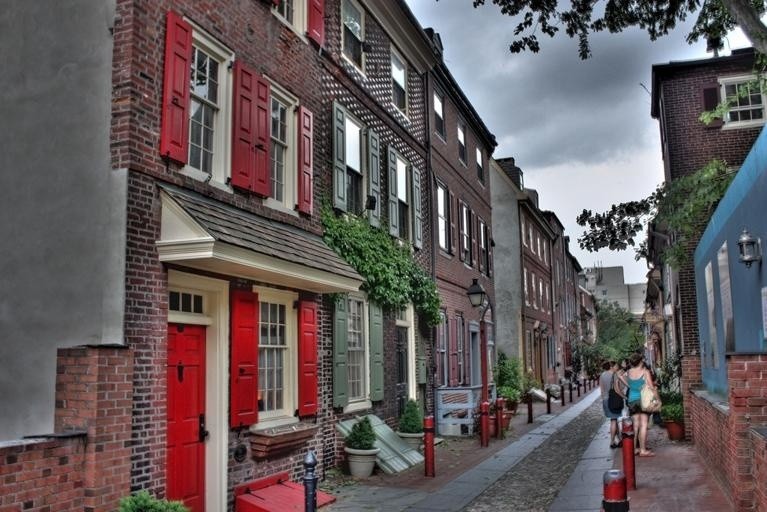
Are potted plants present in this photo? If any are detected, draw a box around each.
[342,415,382,477]
[494,351,523,416]
[397,398,426,452]
[520,371,537,403]
[662,399,685,441]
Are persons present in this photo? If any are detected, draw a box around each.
[599,354,661,456]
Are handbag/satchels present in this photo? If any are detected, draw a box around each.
[640,385,662,411]
[609,389,624,413]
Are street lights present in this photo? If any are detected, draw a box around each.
[463,277,491,401]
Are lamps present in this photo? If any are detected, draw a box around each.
[737,227,762,271]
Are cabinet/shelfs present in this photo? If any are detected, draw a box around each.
[434,384,497,436]
